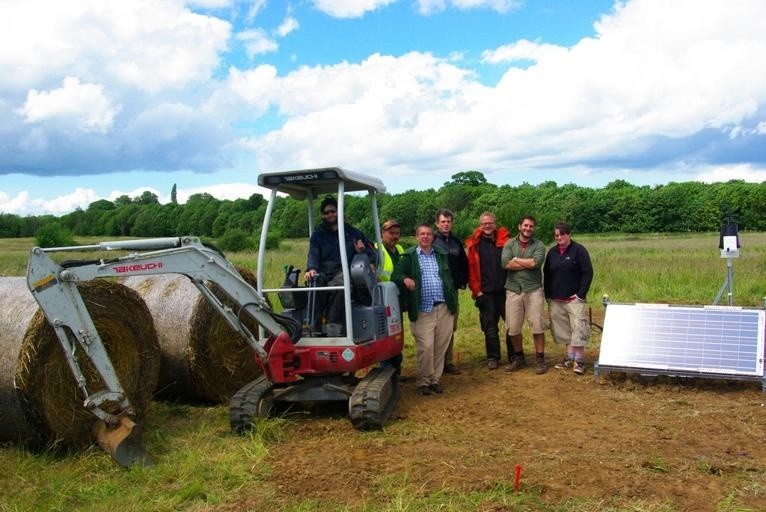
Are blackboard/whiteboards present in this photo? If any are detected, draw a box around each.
[26,165,405,471]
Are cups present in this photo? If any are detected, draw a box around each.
[323,209,336,215]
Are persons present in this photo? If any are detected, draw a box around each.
[390,223,459,395]
[433,208,468,374]
[373,219,408,382]
[543,223,593,374]
[501,215,547,374]
[464,211,515,369]
[304,198,379,334]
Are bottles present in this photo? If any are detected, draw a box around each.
[382,219,400,229]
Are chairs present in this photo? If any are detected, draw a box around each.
[487,358,500,370]
[573,358,585,374]
[430,383,444,393]
[505,354,528,372]
[554,358,572,370]
[417,384,431,395]
[443,363,461,375]
[534,357,547,375]
